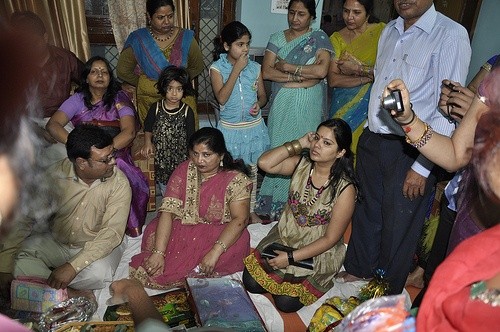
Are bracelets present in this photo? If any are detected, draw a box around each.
[283,139,302,157]
[151,247,167,258]
[392,101,433,149]
[214,240,227,252]
[287,65,303,82]
[359,65,371,76]
[287,251,294,266]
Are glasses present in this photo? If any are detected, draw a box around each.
[88,147,120,165]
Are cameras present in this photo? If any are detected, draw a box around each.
[381,89,403,113]
[445,83,462,108]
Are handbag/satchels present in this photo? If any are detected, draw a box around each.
[261,242,313,270]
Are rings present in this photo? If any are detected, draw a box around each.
[200,266,202,270]
[148,266,151,270]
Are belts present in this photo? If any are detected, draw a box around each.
[367,127,406,142]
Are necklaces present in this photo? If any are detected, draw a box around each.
[288,27,310,41]
[349,29,353,44]
[149,26,173,42]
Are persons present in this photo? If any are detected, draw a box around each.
[380,68,500,332]
[14,11,86,146]
[44,56,150,238]
[128,126,255,290]
[437,54,500,131]
[336,0,472,302]
[0,0,36,332]
[117,0,204,212]
[141,66,196,201]
[262,0,329,155]
[209,20,271,225]
[10,125,133,290]
[242,117,361,315]
[328,0,386,170]
